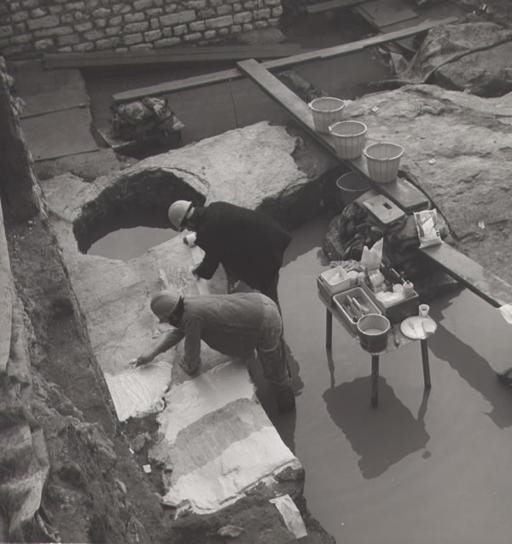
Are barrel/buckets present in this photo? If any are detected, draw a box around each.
[356,314,390,354]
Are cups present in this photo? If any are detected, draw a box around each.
[418,303,430,318]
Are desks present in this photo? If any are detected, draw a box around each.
[318,262,437,407]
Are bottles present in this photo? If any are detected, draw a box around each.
[402,278,414,298]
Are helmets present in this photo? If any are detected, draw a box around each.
[150,290,180,324]
[168,200,192,232]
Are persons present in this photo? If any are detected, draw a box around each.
[167,196,294,382]
[134,288,298,422]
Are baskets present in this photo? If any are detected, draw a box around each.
[308,96,404,202]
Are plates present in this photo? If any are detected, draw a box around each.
[399,315,438,341]
